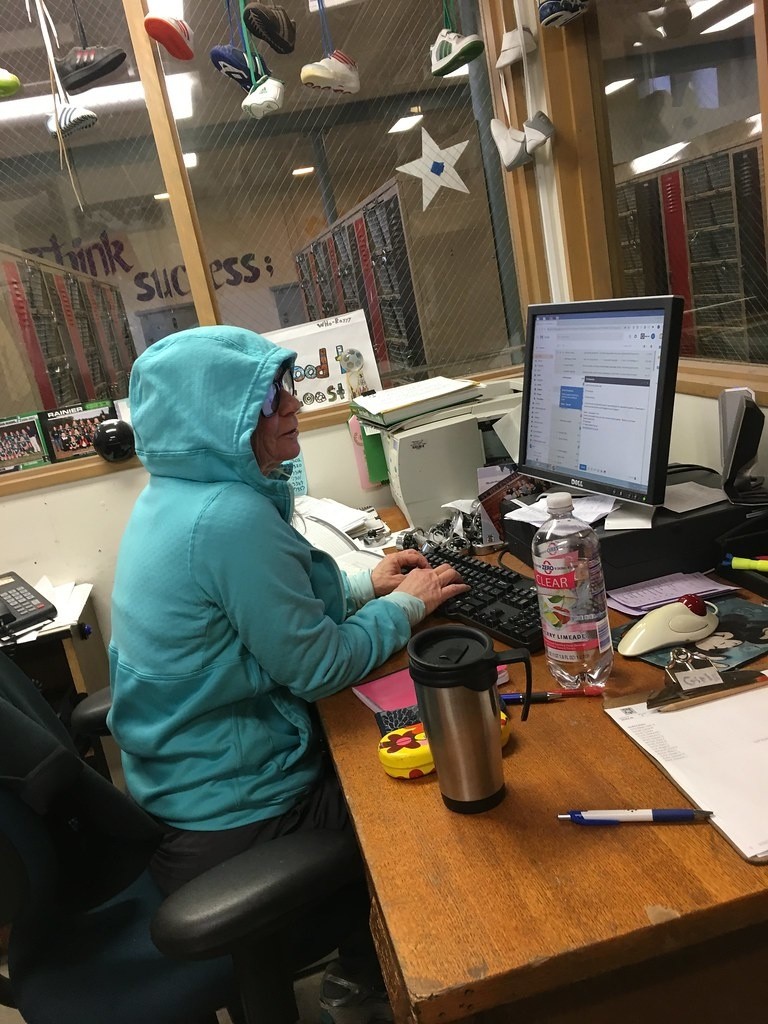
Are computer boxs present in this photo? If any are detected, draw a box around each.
[501,466,768,598]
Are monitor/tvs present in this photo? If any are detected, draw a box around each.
[517,295,684,508]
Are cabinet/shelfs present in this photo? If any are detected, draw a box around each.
[0,244,138,420]
[296,120,512,389]
[613,113,768,363]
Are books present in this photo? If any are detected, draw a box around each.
[349,377,488,434]
[353,655,510,715]
[289,494,390,574]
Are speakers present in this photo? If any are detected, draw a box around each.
[718,386,768,505]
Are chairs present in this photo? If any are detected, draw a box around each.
[0,649,371,1024]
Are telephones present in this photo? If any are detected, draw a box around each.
[0,571,57,638]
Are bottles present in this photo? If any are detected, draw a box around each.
[531,492,615,689]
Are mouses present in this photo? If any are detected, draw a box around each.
[617,594,718,657]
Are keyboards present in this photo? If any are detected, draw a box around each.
[400,544,545,655]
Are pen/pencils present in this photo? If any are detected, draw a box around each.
[557,808,713,824]
[500,692,565,704]
[550,686,602,697]
[657,680,768,713]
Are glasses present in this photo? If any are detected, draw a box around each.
[260,368,296,417]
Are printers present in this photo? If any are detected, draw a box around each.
[380,378,526,544]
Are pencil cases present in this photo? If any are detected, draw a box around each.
[377,711,510,779]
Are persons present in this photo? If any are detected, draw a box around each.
[51,414,106,452]
[103,325,472,1024]
[0,425,41,461]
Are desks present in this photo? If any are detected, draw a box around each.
[319,504,768,1024]
[0,592,129,800]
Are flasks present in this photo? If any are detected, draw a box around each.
[407,625,532,815]
